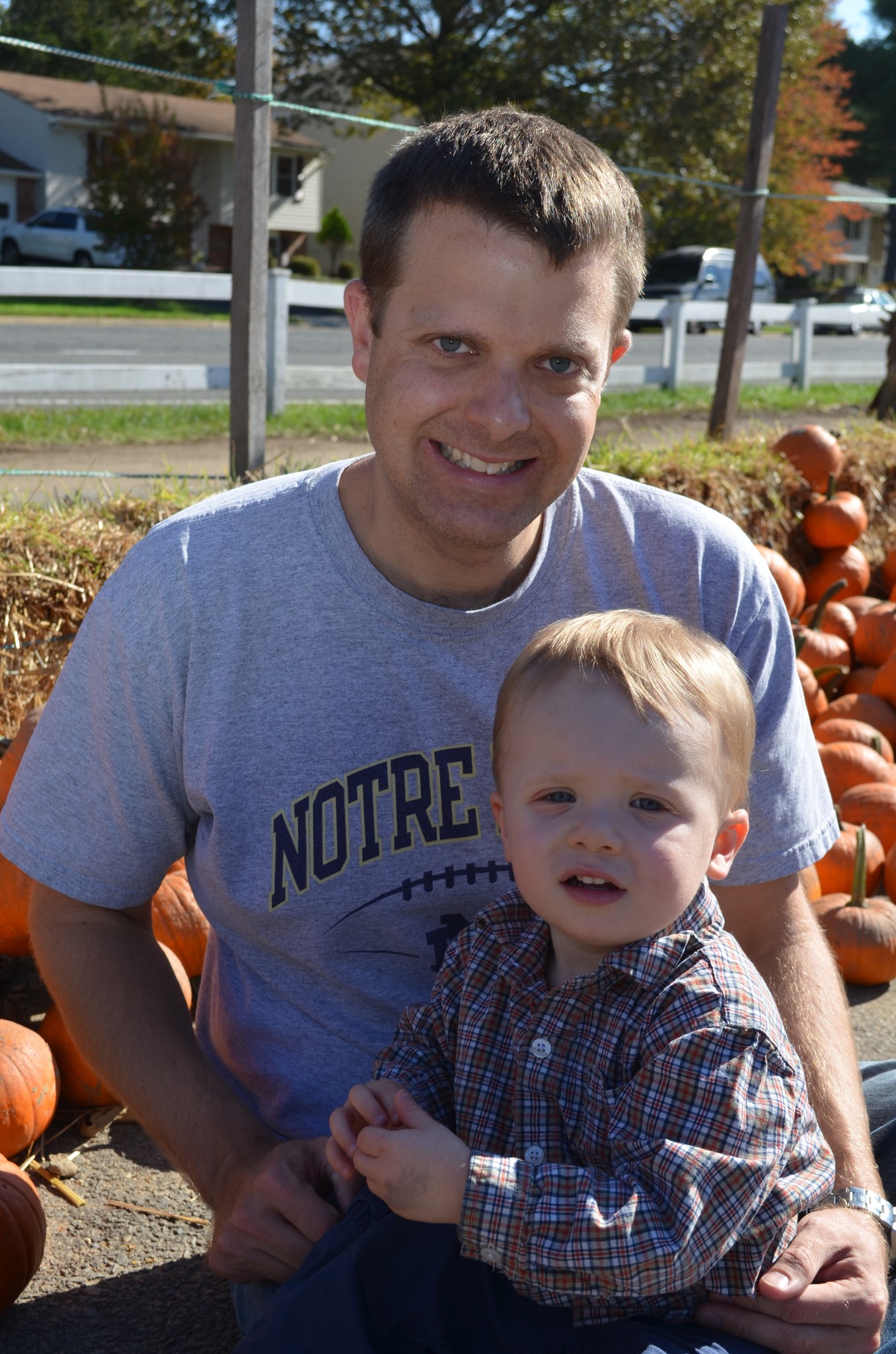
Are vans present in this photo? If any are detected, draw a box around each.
[629,245,776,334]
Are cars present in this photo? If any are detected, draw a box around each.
[817,286,896,334]
[1,205,127,268]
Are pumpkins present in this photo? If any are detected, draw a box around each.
[0,705,210,1312]
[749,424,896,984]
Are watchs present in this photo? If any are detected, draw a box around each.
[827,1187,896,1268]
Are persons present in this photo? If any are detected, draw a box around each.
[324,608,837,1354]
[0,104,896,1354]
